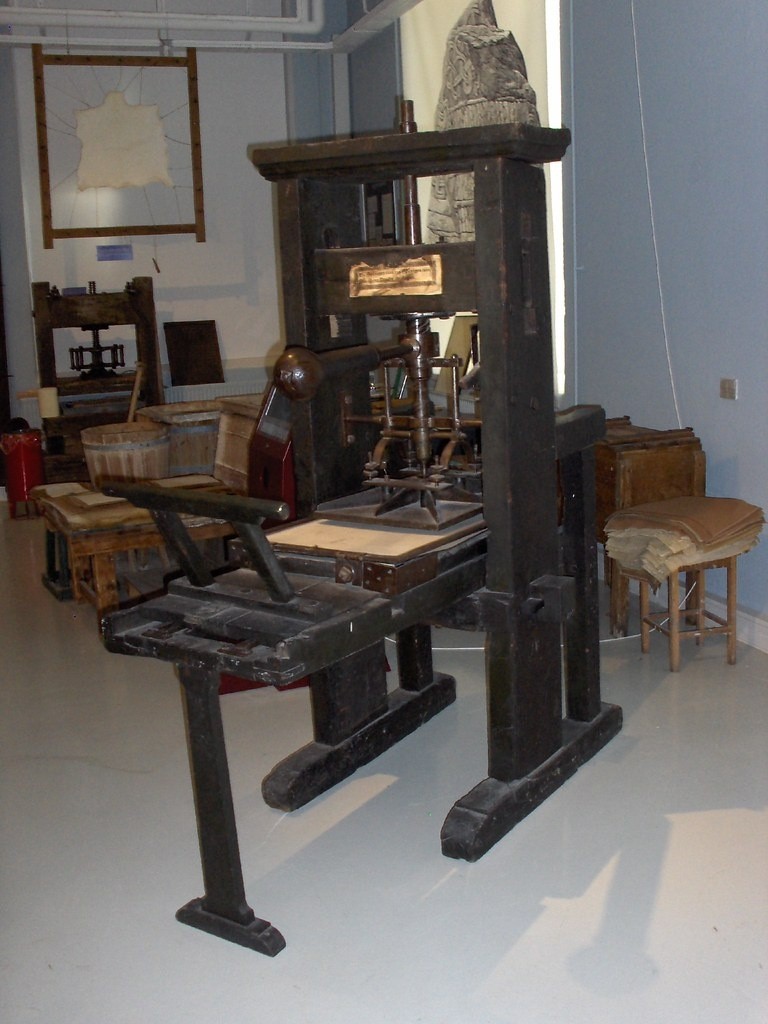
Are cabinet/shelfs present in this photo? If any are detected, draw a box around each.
[558,415,706,639]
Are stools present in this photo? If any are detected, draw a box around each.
[638,555,737,672]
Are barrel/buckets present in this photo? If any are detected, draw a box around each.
[81,422,172,491]
[134,400,221,480]
[213,393,269,496]
